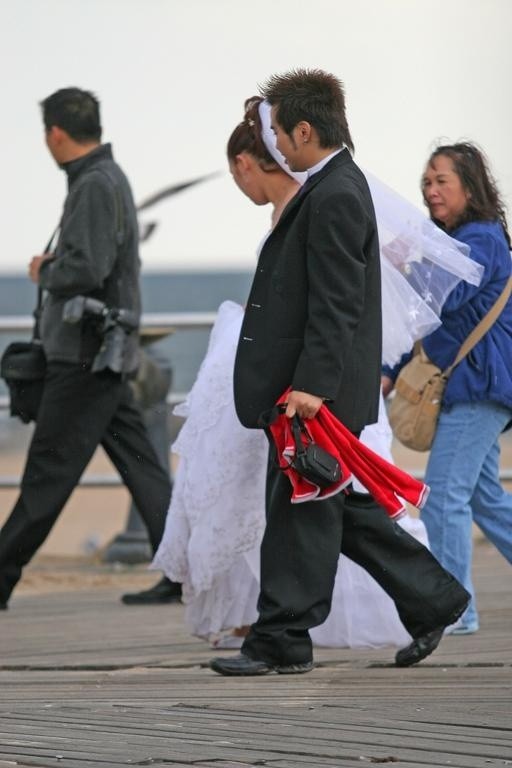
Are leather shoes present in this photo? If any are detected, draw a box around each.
[121,572,186,605]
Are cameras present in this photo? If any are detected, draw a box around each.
[61,293,142,379]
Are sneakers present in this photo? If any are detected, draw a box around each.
[208,645,313,676]
[395,594,469,668]
[444,617,481,635]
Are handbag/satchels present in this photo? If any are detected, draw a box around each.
[386,351,448,452]
[0,342,48,426]
[297,442,341,488]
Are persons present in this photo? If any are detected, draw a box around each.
[209,102,374,652]
[206,64,472,680]
[380,140,512,639]
[0,86,184,608]
